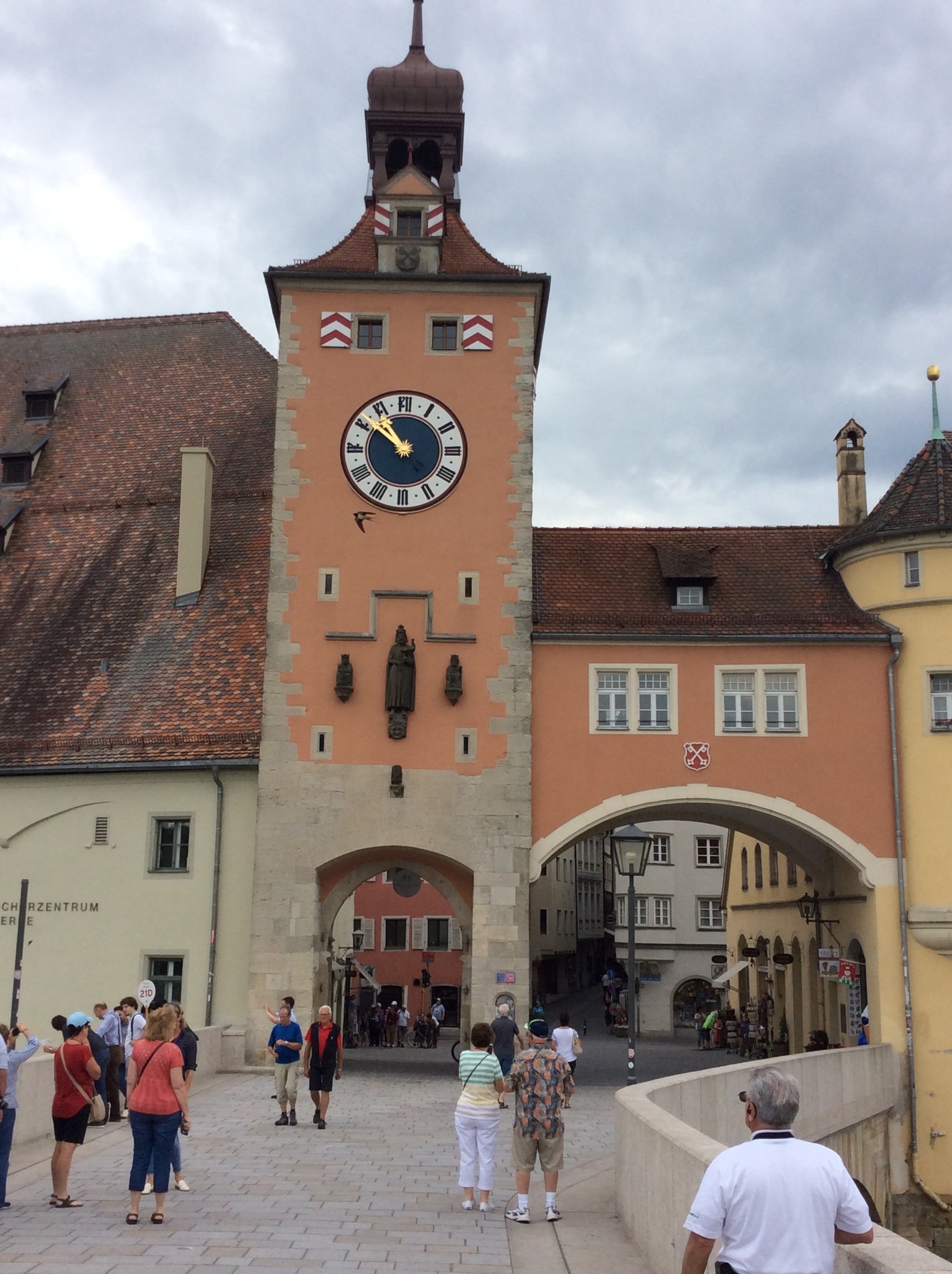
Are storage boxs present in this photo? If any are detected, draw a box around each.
[613,1027,627,1037]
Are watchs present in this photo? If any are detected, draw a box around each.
[337,1068,342,1072]
[48,1046,54,1053]
[285,1041,289,1047]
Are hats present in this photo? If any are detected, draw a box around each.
[437,997,441,1001]
[66,1011,94,1030]
[524,1017,549,1038]
[391,1001,397,1005]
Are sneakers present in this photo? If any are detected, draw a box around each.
[505,1207,530,1223]
[87,1102,111,1128]
[544,1205,563,1222]
[479,1202,494,1212]
[460,1199,474,1210]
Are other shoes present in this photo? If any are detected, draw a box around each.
[313,1109,325,1129]
[275,1112,298,1125]
[560,1096,571,1108]
[357,1040,438,1048]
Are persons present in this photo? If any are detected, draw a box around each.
[360,997,446,1050]
[267,1005,304,1126]
[336,653,354,687]
[43,1014,111,1129]
[454,1021,503,1212]
[694,992,754,1058]
[502,1018,577,1223]
[384,624,416,711]
[123,1006,191,1226]
[112,1005,131,1119]
[550,1011,581,1108]
[860,1005,870,1046]
[445,654,462,689]
[680,1064,874,1274]
[300,1004,343,1130]
[263,996,297,1100]
[602,971,622,1035]
[139,1002,198,1194]
[93,1001,124,1123]
[119,997,148,1081]
[489,1004,525,1109]
[0,1019,41,1210]
[48,1011,102,1209]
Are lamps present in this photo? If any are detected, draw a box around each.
[337,929,366,963]
[796,893,840,948]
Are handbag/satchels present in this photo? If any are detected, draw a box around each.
[573,1037,583,1055]
[88,1094,106,1123]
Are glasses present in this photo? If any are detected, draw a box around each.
[737,1090,749,1102]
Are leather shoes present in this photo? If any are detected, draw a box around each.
[141,1182,154,1194]
[174,1179,190,1191]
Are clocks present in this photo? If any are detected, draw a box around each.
[339,389,468,516]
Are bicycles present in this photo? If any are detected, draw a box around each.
[407,1019,435,1048]
[450,1038,460,1062]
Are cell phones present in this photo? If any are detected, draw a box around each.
[12,1027,20,1036]
[486,1043,493,1056]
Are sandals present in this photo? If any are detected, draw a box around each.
[125,1212,138,1224]
[49,1194,84,1208]
[151,1211,164,1224]
[497,1098,508,1109]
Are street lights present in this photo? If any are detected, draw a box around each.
[611,822,654,1085]
